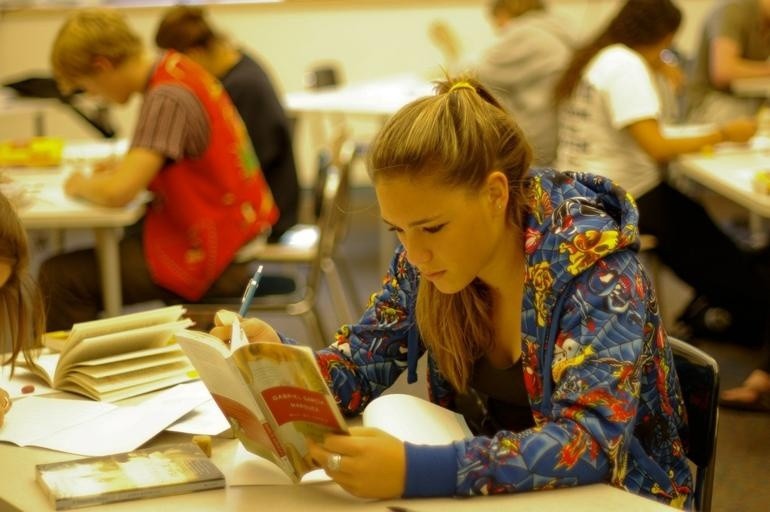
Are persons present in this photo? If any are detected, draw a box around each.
[714,364,770,414]
[679,0,770,130]
[209,78,695,508]
[556,0,767,362]
[156,4,300,242]
[39,7,281,335]
[0,192,47,428]
[429,1,571,166]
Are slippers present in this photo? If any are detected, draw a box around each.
[719,383,768,411]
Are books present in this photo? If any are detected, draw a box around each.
[174,309,350,481]
[24,303,206,405]
[35,443,224,509]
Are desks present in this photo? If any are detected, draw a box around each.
[671,127,770,250]
[1,142,156,318]
[284,80,438,218]
[1,343,679,511]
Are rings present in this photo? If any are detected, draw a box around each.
[3,396,9,411]
[327,452,343,479]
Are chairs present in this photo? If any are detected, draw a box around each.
[665,336,723,512]
[178,162,343,346]
[324,141,366,330]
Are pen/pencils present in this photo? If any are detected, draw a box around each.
[239,265,263,318]
